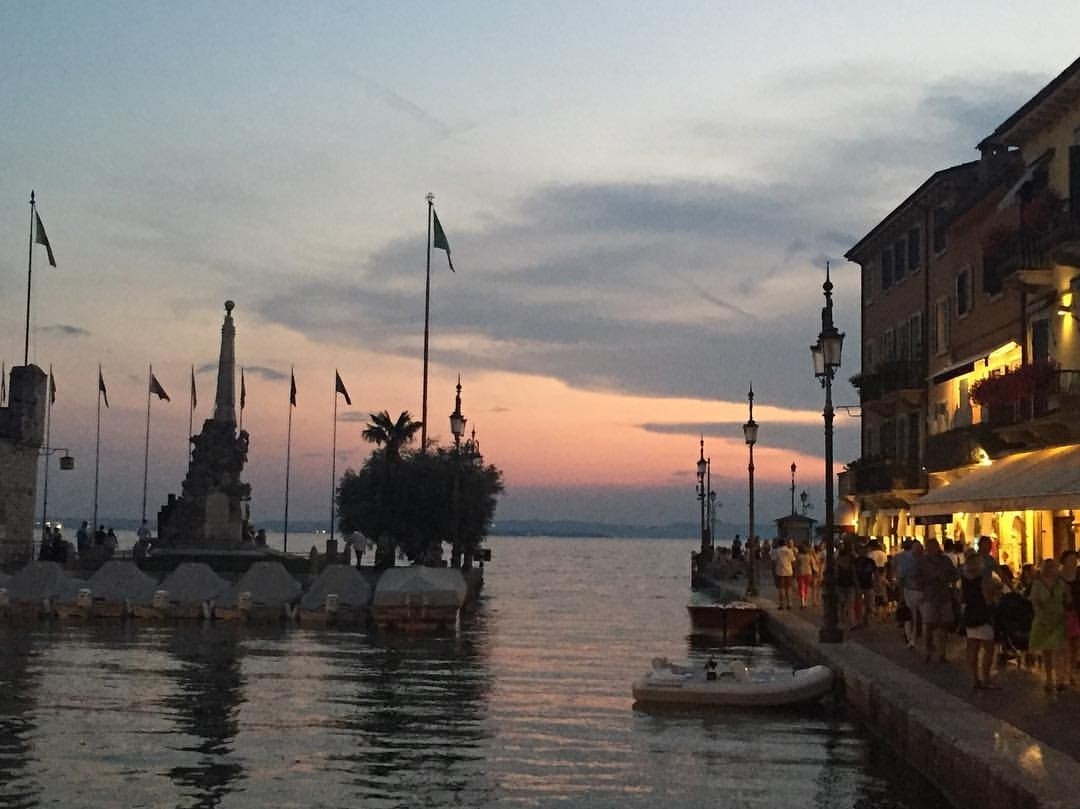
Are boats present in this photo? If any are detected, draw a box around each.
[631,657,831,707]
[685,603,763,631]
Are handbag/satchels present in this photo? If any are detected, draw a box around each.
[896,602,913,622]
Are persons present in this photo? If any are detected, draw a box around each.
[734,534,1079,696]
[247,525,368,569]
[39,520,153,563]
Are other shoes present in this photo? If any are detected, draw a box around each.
[1056,683,1064,692]
[804,605,807,608]
[923,656,929,665]
[800,606,803,610]
[778,606,784,609]
[907,641,916,648]
[1045,684,1051,691]
[961,674,979,688]
[786,606,791,609]
[983,678,999,689]
[937,656,950,665]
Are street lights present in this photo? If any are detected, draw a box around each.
[800,493,808,515]
[811,323,846,642]
[696,459,717,562]
[791,464,796,514]
[42,446,74,543]
[743,420,761,596]
[449,412,467,569]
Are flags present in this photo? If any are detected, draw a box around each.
[151,374,170,401]
[2,368,5,402]
[433,210,456,273]
[337,372,351,405]
[240,376,246,408]
[100,374,109,408]
[192,374,197,408]
[290,375,297,407]
[51,374,56,403]
[36,214,56,268]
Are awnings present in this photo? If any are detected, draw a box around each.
[909,445,1080,517]
